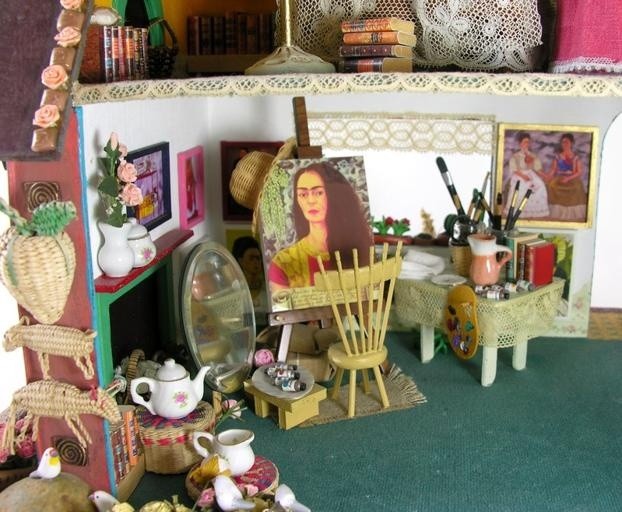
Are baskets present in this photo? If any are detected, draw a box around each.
[116,350,160,405]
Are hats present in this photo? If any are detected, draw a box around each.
[229,136,297,240]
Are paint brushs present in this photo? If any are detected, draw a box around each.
[435,157,534,236]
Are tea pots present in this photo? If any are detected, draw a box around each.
[131,358,210,419]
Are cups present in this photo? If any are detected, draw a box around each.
[468,233,514,287]
[194,429,256,476]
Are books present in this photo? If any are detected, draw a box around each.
[141,28,147,69]
[524,241,555,288]
[338,44,413,58]
[341,17,415,34]
[133,28,140,79]
[112,25,119,82]
[506,233,538,284]
[343,32,417,46]
[516,238,547,282]
[125,26,134,80]
[138,28,144,74]
[77,25,112,84]
[186,12,276,57]
[118,26,126,81]
[337,57,413,74]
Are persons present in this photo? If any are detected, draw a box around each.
[268,163,375,295]
[539,133,586,220]
[503,130,549,217]
[228,235,268,306]
